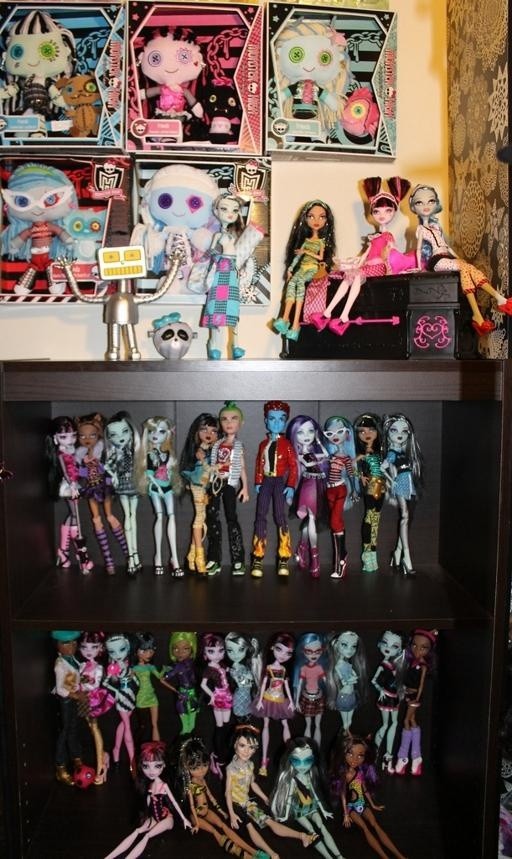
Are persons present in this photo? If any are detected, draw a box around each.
[196,193,247,360]
[353,413,388,572]
[103,633,140,783]
[51,630,83,786]
[103,411,143,574]
[372,627,404,775]
[225,727,323,859]
[226,630,259,723]
[310,175,407,333]
[48,417,94,573]
[324,416,360,578]
[289,415,329,578]
[292,633,325,746]
[272,734,346,859]
[126,1,263,158]
[179,738,271,859]
[326,630,367,740]
[379,413,423,573]
[99,741,192,858]
[160,632,199,744]
[204,401,249,575]
[264,2,399,157]
[1,155,112,302]
[411,185,512,334]
[55,246,187,360]
[393,627,436,776]
[255,634,293,776]
[180,414,233,571]
[251,401,298,575]
[1,2,126,151]
[76,413,130,575]
[76,632,110,786]
[141,417,184,578]
[332,728,407,859]
[273,200,335,339]
[131,633,179,743]
[200,633,232,764]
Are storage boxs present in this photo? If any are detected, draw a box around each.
[283,271,489,360]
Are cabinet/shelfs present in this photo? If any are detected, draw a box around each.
[1,359,512,858]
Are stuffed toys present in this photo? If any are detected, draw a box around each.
[134,161,271,305]
